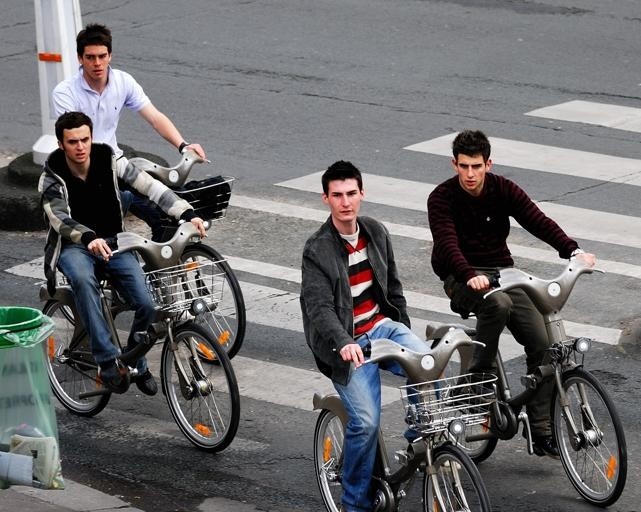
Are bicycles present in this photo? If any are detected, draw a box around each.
[427,259,628,506]
[50,147,246,365]
[40,223,241,453]
[312,330,499,511]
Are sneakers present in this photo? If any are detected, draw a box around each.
[406,444,455,467]
[136,368,159,396]
[100,362,131,394]
[466,366,495,403]
[522,425,561,458]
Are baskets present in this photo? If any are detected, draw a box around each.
[141,258,226,313]
[398,373,498,435]
[159,174,235,227]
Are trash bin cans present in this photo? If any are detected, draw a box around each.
[0,305,66,491]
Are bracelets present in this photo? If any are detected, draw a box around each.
[178,142,189,151]
[570,249,584,256]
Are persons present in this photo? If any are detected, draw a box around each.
[52,21,207,281]
[38,110,206,396]
[426,128,597,460]
[296,160,442,512]
